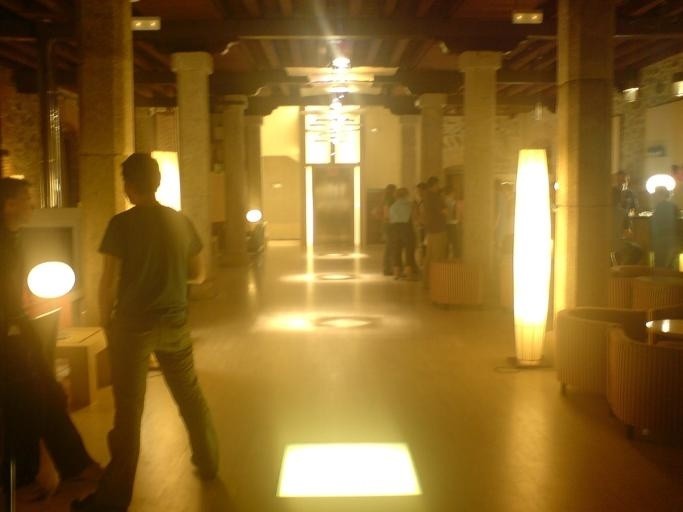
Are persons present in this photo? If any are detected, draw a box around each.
[69,152,221,511]
[611,164,683,271]
[381,175,462,282]
[1,173,105,501]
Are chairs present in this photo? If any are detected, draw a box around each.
[429,258,478,307]
[555,264,683,440]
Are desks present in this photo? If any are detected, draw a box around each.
[53,327,108,406]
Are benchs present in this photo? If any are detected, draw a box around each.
[247,221,267,256]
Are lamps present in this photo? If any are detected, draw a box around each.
[672,72,683,84]
[513,148,551,366]
[621,67,640,93]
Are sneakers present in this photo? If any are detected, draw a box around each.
[71,497,127,512]
[60,462,108,486]
